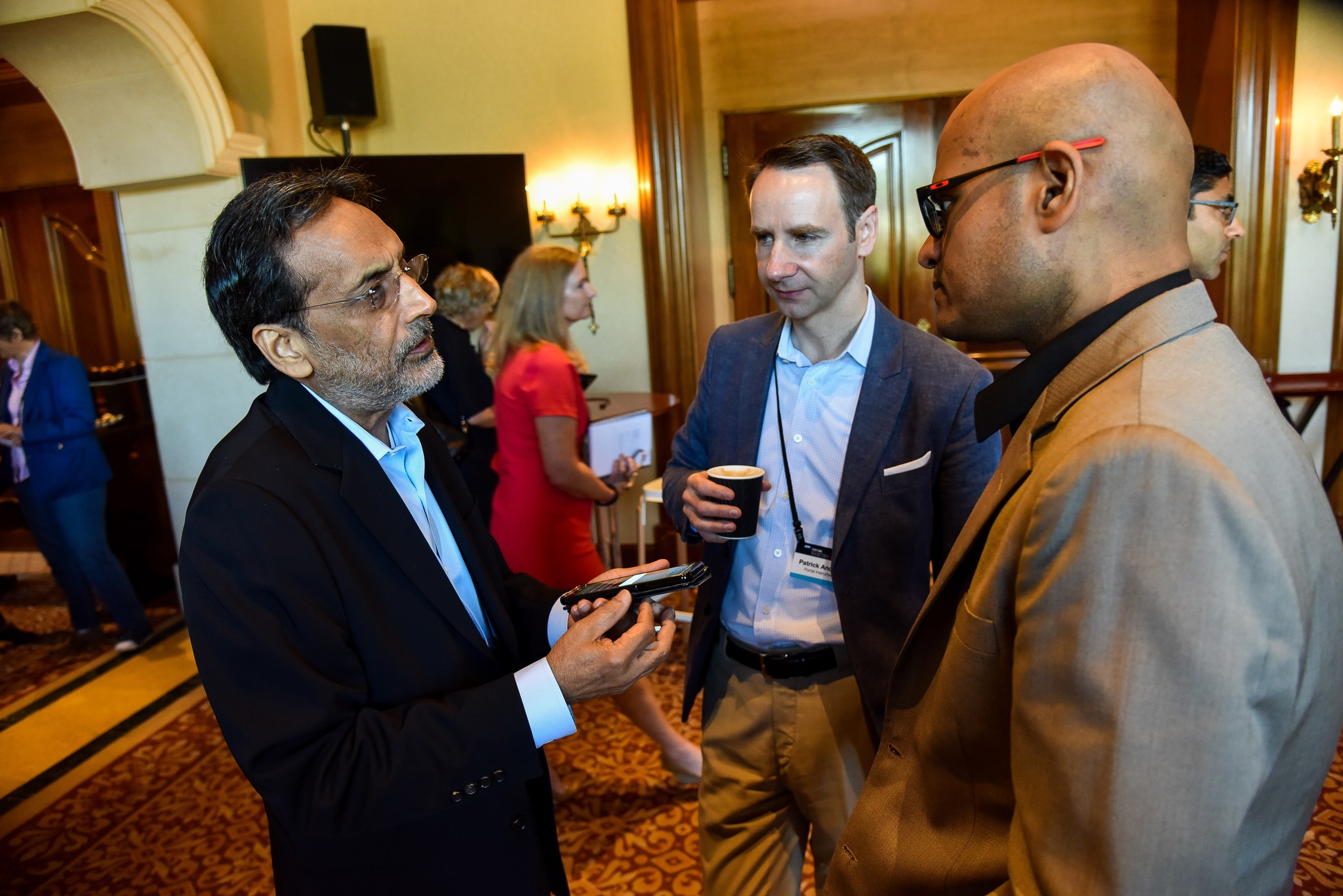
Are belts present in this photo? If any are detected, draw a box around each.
[721,636,853,680]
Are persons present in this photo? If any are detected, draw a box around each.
[180,170,676,895]
[0,300,153,653]
[424,244,704,784]
[826,43,1341,896]
[662,135,1004,895]
[1188,145,1245,281]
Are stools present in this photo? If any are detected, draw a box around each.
[637,477,692,644]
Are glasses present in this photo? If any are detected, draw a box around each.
[1189,198,1240,225]
[914,137,1108,238]
[297,252,428,311]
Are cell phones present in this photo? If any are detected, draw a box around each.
[561,561,705,605]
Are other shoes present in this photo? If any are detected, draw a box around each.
[0,572,15,592]
[659,756,701,785]
[115,623,155,653]
[0,623,36,642]
[73,623,103,637]
[550,768,584,803]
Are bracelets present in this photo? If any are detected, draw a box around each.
[595,482,619,507]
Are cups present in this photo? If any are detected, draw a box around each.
[706,465,766,540]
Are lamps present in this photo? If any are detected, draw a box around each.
[524,177,630,337]
[1297,98,1343,229]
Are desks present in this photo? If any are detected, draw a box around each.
[588,390,690,571]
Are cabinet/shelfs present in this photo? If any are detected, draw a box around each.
[87,370,186,610]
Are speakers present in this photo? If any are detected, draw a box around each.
[301,23,379,128]
